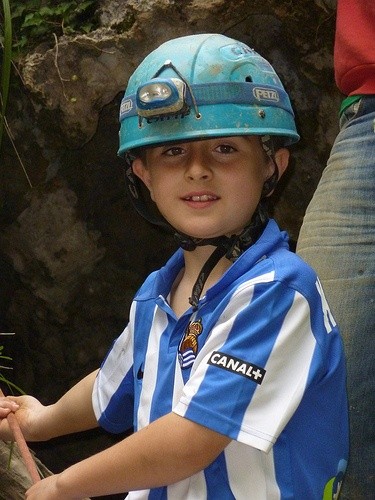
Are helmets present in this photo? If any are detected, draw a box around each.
[117,33,300,160]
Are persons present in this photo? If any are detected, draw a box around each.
[0,33,350,500]
[295,0,375,500]
